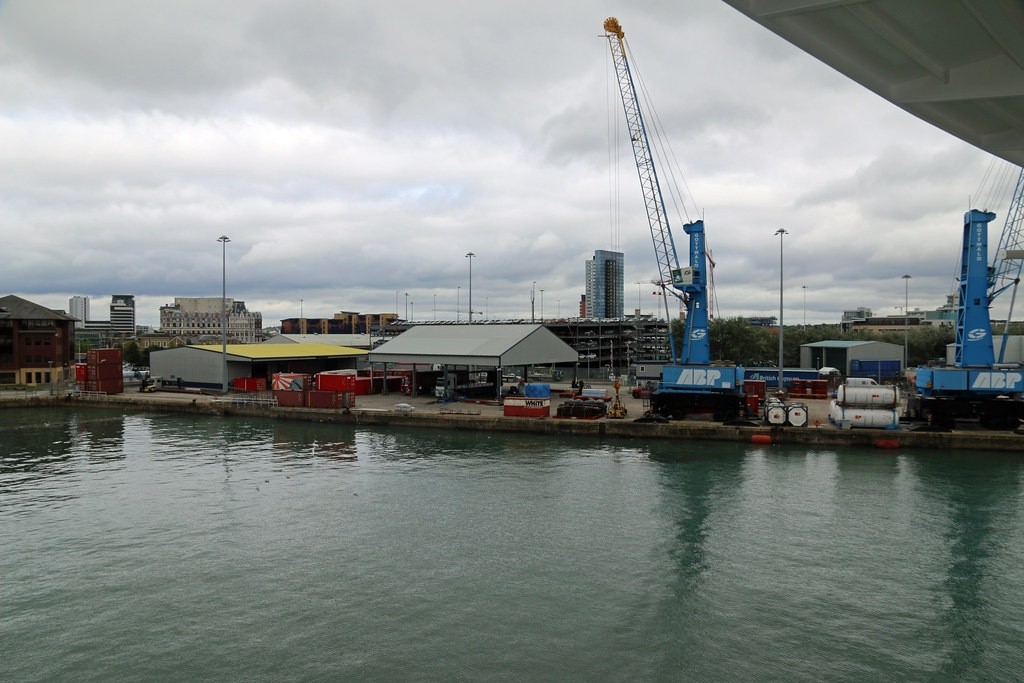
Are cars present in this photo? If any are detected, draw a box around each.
[633,387,650,399]
[391,317,667,381]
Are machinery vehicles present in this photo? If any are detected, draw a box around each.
[140,380,156,393]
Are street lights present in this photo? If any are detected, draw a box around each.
[774,227,788,390]
[540,289,544,323]
[458,286,461,325]
[217,235,231,390]
[901,274,912,368]
[634,281,641,310]
[465,252,476,325]
[404,293,409,320]
[49,361,53,392]
[533,281,536,325]
[802,285,808,331]
[396,290,399,319]
[300,299,303,334]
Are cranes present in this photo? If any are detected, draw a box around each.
[602,17,746,421]
